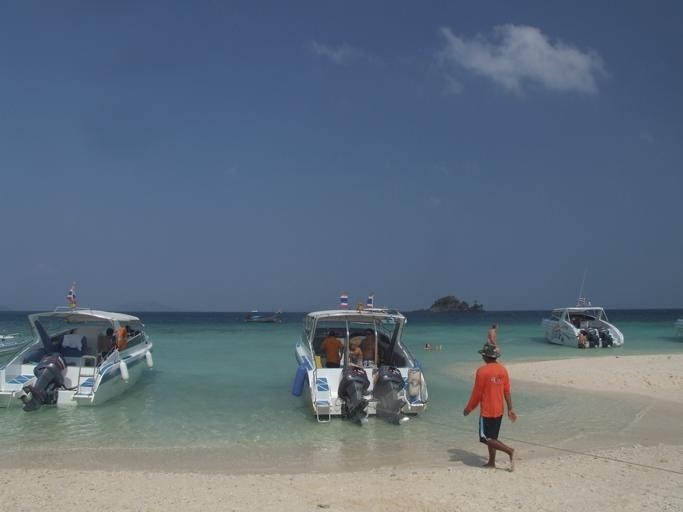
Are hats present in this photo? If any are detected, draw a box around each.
[477,343,503,359]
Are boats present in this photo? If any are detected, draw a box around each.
[245,313,280,324]
[0,279,155,409]
[294,292,430,423]
[0,331,33,357]
[541,282,624,349]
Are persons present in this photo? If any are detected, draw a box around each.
[577,330,587,346]
[361,328,385,362]
[349,336,363,364]
[464,343,517,467]
[320,331,344,368]
[488,323,496,345]
[102,328,114,357]
